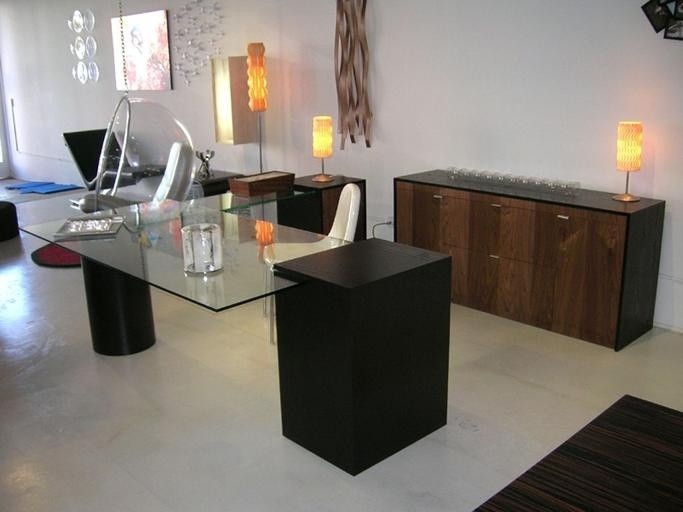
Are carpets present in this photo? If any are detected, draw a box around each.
[469,393,682,511]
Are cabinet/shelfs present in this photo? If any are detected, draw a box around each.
[393,169,666,352]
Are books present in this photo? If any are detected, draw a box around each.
[53,212,126,244]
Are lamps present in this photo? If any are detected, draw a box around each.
[640,1,683,42]
[245,42,268,220]
[311,116,335,183]
[612,122,643,202]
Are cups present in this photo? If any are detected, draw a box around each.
[447,166,575,198]
[180,223,223,275]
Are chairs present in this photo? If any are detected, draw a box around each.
[61,93,243,235]
